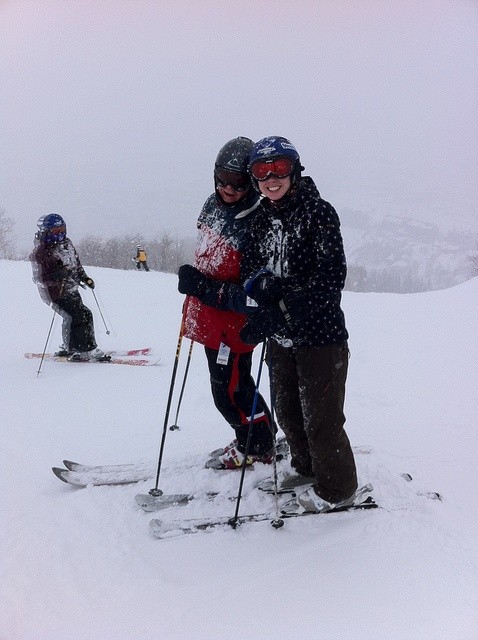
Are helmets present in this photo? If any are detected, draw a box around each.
[248,136,300,193]
[213,137,255,194]
[43,214,67,240]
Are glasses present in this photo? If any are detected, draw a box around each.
[250,155,294,181]
[213,168,250,192]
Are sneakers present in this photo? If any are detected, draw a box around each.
[209,439,238,458]
[260,467,315,490]
[205,446,274,470]
[280,487,363,516]
[68,347,111,361]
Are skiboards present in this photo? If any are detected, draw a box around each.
[51,435,292,487]
[133,473,443,539]
[25,346,162,365]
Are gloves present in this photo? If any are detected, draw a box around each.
[179,263,205,295]
[239,305,288,344]
[256,276,292,299]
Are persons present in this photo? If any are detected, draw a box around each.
[29,213,111,362]
[239,134,359,515]
[132,245,150,272]
[177,136,279,467]
